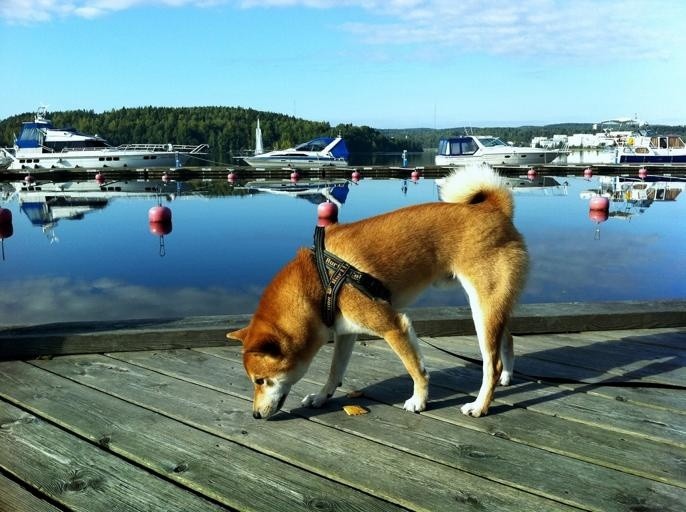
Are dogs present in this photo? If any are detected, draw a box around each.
[226,162,531,420]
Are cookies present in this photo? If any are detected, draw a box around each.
[341,405,369,416]
[345,390,363,398]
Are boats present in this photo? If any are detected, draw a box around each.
[593,118,685,162]
[0,178,199,246]
[231,179,351,214]
[231,129,350,168]
[433,120,573,165]
[578,174,686,220]
[496,174,571,200]
[0,105,213,167]
